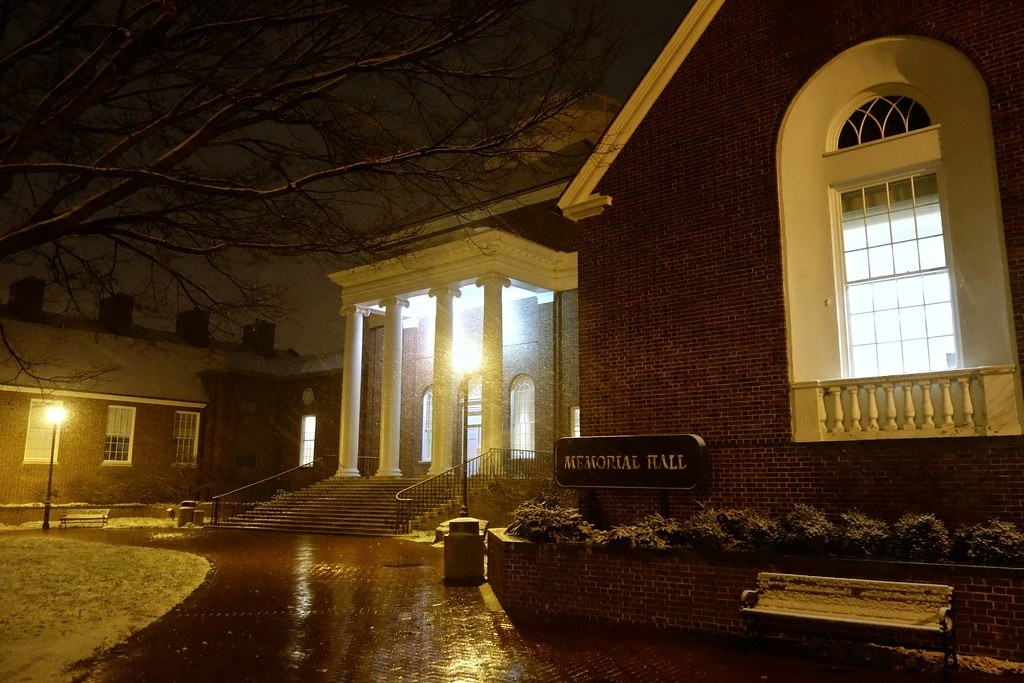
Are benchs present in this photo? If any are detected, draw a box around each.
[740,572,958,682]
[60,509,111,528]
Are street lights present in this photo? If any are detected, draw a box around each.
[42,405,68,530]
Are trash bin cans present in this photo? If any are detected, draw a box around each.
[177,500,202,529]
[444,521,486,585]
[193,509,205,526]
